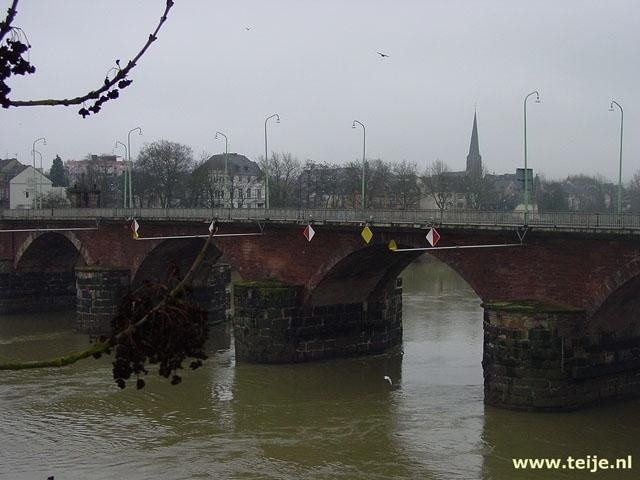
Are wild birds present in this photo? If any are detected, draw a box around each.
[384,375,393,385]
[376,51,389,58]
[245,27,253,30]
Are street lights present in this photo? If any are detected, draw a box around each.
[524,90,540,225]
[610,99,624,221]
[32,137,47,213]
[352,119,366,216]
[115,126,142,210]
[216,131,228,210]
[264,113,280,213]
